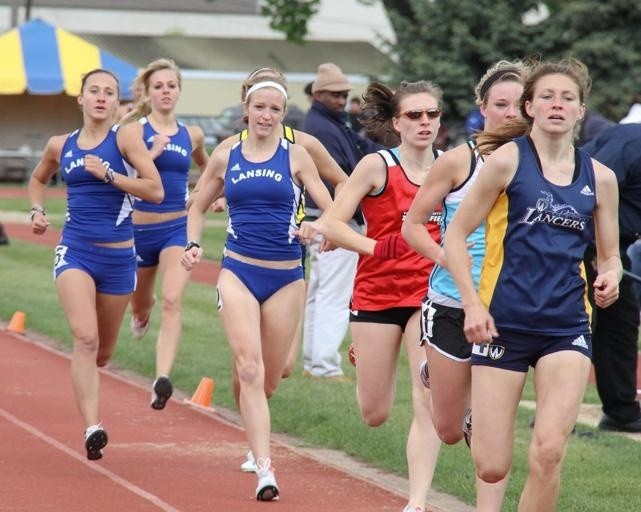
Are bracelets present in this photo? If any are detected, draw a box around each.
[30,206,47,222]
[104,166,114,182]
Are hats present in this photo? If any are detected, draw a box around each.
[310,60,355,97]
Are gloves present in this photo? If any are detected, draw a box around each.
[373,233,410,261]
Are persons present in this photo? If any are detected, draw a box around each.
[401,48,640,512]
[119,57,227,411]
[27,67,166,462]
[180,56,447,511]
[115,75,150,125]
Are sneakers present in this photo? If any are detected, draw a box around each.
[130,292,156,339]
[596,414,641,432]
[241,448,257,473]
[255,466,279,502]
[418,359,434,390]
[149,372,173,410]
[463,406,473,450]
[85,423,107,460]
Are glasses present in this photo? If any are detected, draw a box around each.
[395,108,444,121]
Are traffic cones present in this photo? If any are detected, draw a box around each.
[3,311,26,335]
[184,378,218,409]
